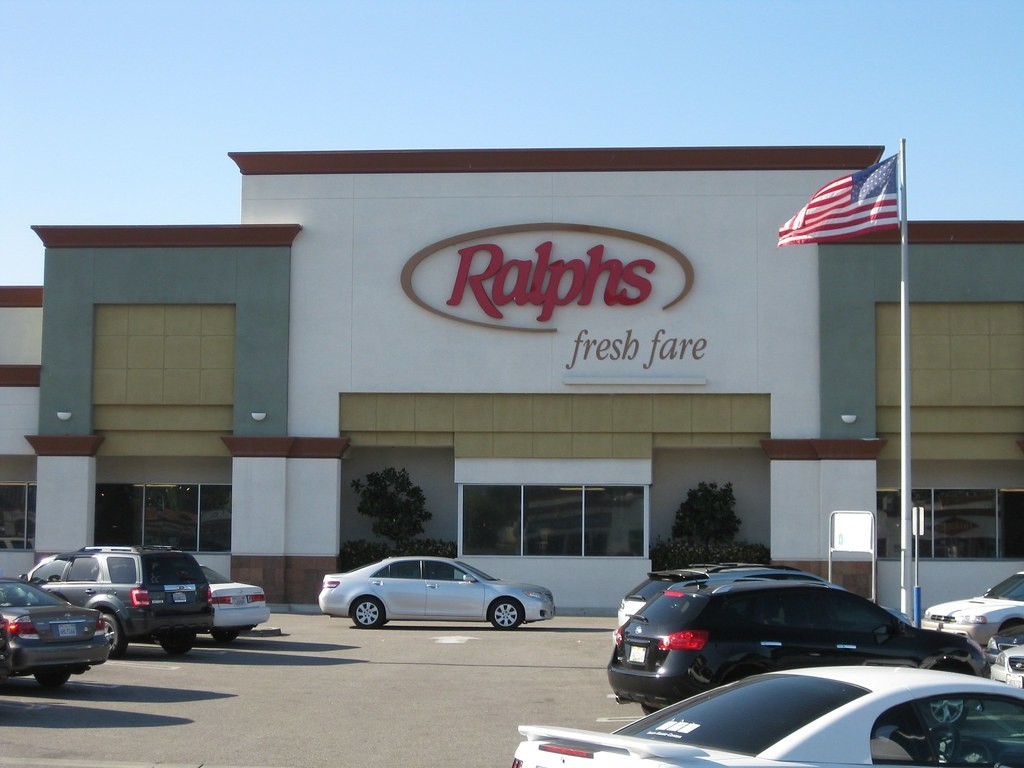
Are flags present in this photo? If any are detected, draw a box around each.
[776,153,898,249]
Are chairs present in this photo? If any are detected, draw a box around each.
[890,729,931,761]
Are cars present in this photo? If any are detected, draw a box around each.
[983,624,1024,679]
[607,578,989,731]
[199,564,271,642]
[990,645,1024,688]
[510,665,1024,768]
[318,555,540,628]
[0,497,35,537]
[923,571,1024,651]
[0,537,35,550]
[0,577,110,688]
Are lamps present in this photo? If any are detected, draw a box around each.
[57,411,71,420]
[842,415,856,424]
[251,412,267,421]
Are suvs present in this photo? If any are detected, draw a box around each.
[612,562,913,648]
[18,545,216,652]
